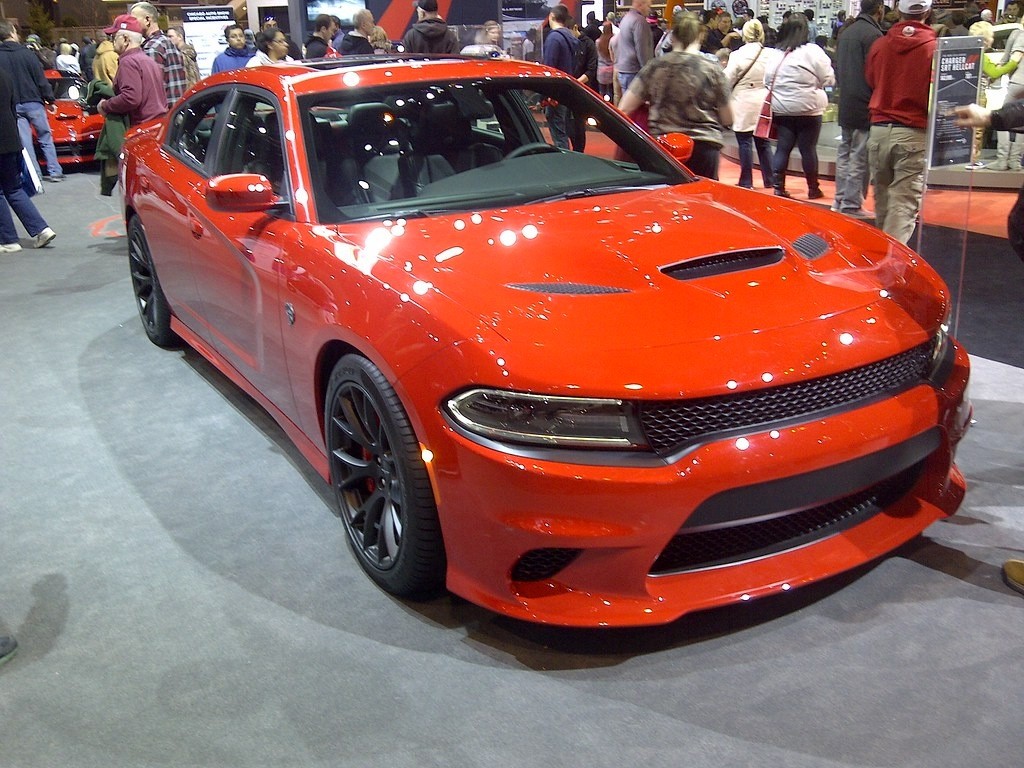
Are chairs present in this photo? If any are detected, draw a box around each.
[266,111,365,208]
[398,101,502,198]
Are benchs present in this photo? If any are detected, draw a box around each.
[196,102,419,167]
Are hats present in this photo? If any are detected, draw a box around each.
[83,37,92,44]
[899,0,932,14]
[244,29,253,39]
[740,8,754,17]
[413,0,438,12]
[103,14,143,35]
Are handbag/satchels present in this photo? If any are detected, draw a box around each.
[752,91,779,142]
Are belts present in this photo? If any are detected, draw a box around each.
[874,121,910,127]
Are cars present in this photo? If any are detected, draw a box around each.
[30,70,105,167]
[117,53,972,630]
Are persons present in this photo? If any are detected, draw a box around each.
[212,9,388,112]
[401,0,461,56]
[0,2,202,252]
[478,20,501,45]
[536,5,596,154]
[584,0,1024,262]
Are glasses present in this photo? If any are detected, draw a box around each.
[273,39,287,46]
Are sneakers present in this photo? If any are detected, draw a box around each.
[0,242,23,253]
[33,227,56,248]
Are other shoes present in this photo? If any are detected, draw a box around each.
[1007,160,1021,171]
[774,189,790,197]
[0,636,18,666]
[843,209,876,219]
[966,161,985,169]
[985,160,1008,170]
[808,189,824,198]
[51,174,67,182]
[831,207,840,212]
[1001,559,1024,594]
